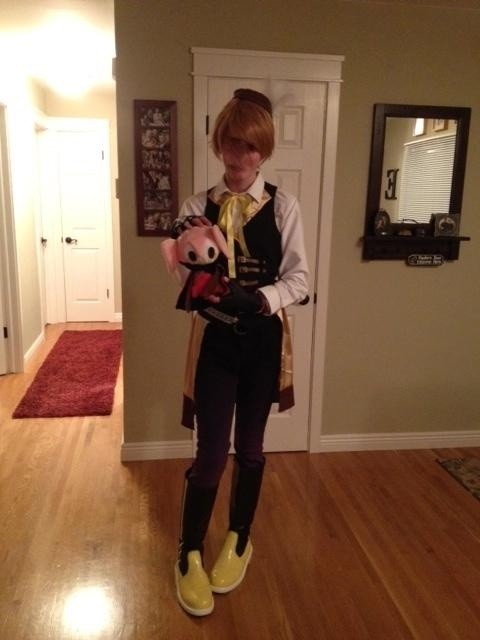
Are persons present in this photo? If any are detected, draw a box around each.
[174,88,311,619]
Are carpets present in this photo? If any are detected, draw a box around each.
[434,455,480,507]
[10,329,127,420]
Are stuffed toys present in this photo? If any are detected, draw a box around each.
[161,223,235,311]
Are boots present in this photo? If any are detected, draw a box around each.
[209,451,267,596]
[173,471,219,621]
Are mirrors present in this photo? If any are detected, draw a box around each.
[354,103,469,267]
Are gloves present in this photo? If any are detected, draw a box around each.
[168,209,211,238]
[210,276,263,321]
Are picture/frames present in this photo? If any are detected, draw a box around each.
[431,118,448,132]
[133,98,180,240]
[430,211,462,238]
